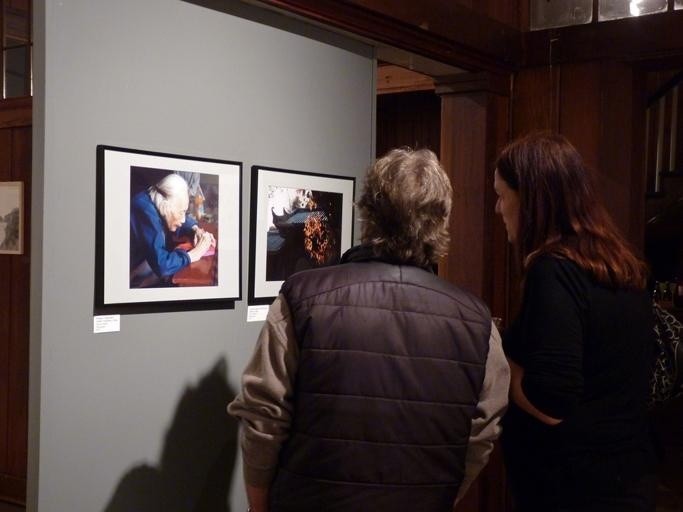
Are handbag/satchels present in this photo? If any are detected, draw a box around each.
[644,302,683,409]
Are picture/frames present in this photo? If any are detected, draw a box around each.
[0,181,25,254]
[247,165,357,306]
[96,146,242,312]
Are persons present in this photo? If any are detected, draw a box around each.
[174,171,205,214]
[227,145,512,512]
[485,132,660,512]
[130,173,218,277]
